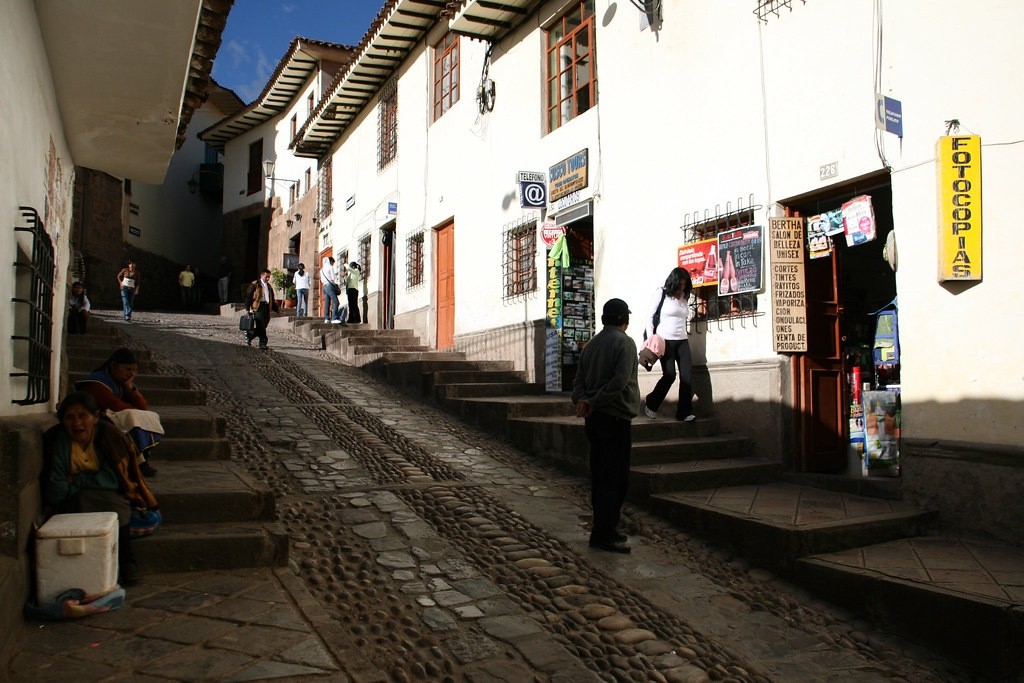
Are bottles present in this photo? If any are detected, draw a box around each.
[719,250,738,294]
[704,241,717,283]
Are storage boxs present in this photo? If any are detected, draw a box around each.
[33,511,119,602]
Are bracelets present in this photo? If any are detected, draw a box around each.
[132,384,136,391]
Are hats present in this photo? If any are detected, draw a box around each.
[603,298,632,318]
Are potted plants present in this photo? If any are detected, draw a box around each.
[270,267,297,308]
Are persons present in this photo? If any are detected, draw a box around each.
[293,263,311,316]
[178,264,201,313]
[342,262,361,324]
[320,257,341,323]
[40,392,160,588]
[72,250,85,283]
[644,267,696,421]
[217,256,231,303]
[571,298,641,553]
[67,282,90,335]
[244,269,279,349]
[72,347,164,477]
[117,259,141,320]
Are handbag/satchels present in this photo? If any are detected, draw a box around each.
[333,284,341,294]
[240,312,253,331]
[644,290,666,341]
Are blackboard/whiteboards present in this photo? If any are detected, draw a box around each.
[718,224,763,296]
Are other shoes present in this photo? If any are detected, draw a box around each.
[125,315,130,320]
[614,533,628,542]
[325,319,330,323]
[331,320,341,323]
[246,334,251,344]
[140,460,158,476]
[590,542,631,553]
[260,345,268,349]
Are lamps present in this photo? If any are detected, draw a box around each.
[262,158,298,182]
[286,219,293,228]
[187,170,224,194]
[295,213,302,222]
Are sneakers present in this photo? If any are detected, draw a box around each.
[644,397,657,419]
[685,415,696,422]
